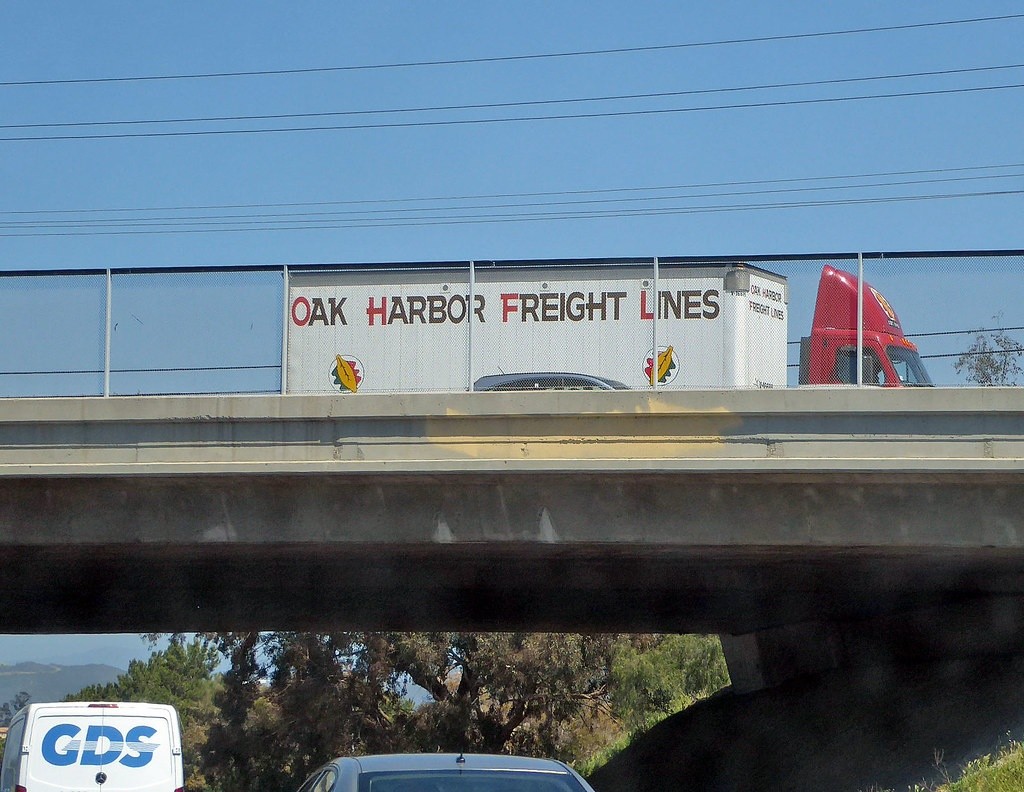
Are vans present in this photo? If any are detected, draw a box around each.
[1,699,185,792]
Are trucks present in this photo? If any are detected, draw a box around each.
[279,252,935,398]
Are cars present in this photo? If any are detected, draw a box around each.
[296,753,595,792]
